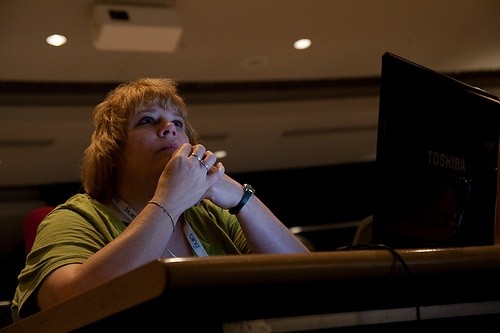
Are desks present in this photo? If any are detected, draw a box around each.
[0,245,500,333]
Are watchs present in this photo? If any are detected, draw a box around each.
[228,184,255,216]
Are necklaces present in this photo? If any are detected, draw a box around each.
[111,192,208,257]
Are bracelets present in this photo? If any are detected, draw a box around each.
[147,201,176,233]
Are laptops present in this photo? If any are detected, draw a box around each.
[338,52,500,250]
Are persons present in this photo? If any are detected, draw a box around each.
[12,80,311,322]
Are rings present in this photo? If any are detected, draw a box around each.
[191,153,201,162]
[200,160,209,171]
